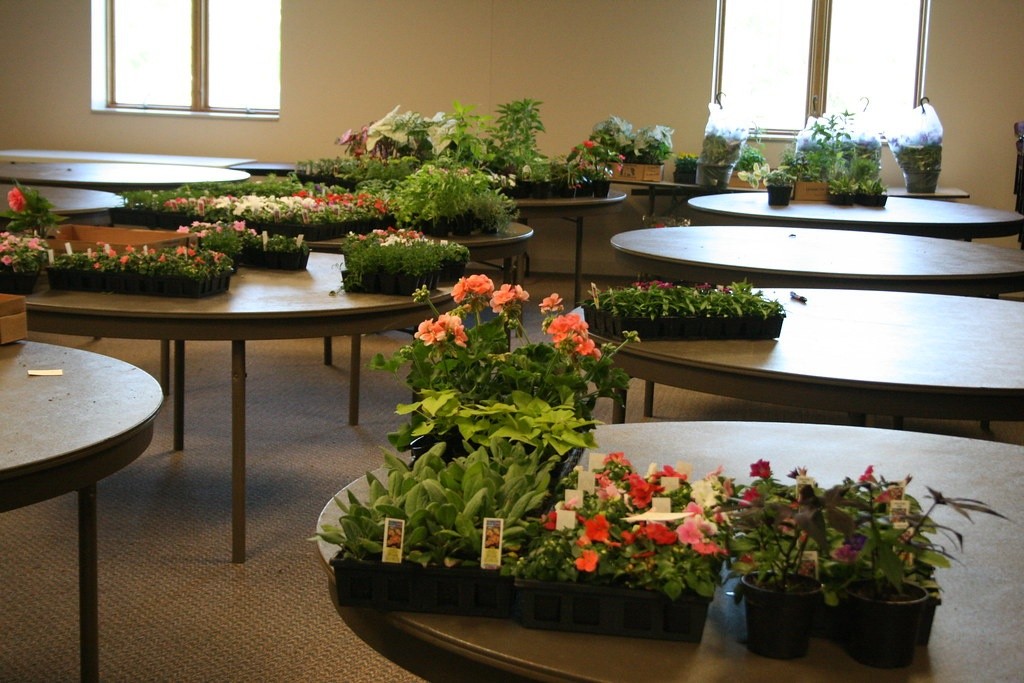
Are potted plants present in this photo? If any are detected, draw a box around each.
[887,129,942,194]
[695,109,888,207]
[293,97,564,199]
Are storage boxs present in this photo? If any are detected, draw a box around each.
[45,219,198,256]
[0,294,28,345]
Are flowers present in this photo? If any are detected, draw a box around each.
[532,474,943,581]
[367,272,641,455]
[673,152,698,172]
[576,275,786,320]
[0,164,520,290]
[566,138,625,188]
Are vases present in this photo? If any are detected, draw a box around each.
[591,181,612,198]
[410,436,587,480]
[581,304,787,345]
[515,555,943,667]
[674,168,697,184]
[0,209,498,298]
[328,552,515,621]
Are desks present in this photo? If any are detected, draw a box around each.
[512,189,627,310]
[305,219,535,366]
[0,341,164,682]
[582,289,1024,432]
[315,424,1024,683]
[0,268,459,564]
[2,147,292,226]
[624,174,1024,239]
[610,227,1024,298]
